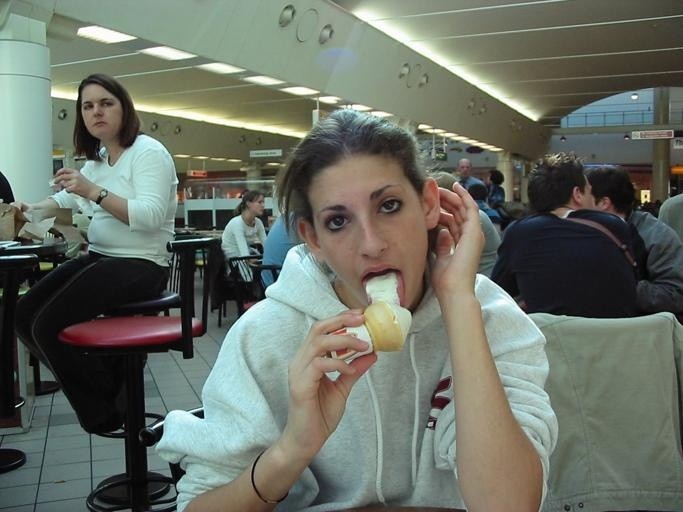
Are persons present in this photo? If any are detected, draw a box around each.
[452,157,485,196]
[467,184,500,225]
[10,73,180,435]
[428,171,502,278]
[221,190,267,311]
[485,170,505,209]
[585,164,683,324]
[488,151,647,319]
[261,206,305,292]
[161,107,560,511]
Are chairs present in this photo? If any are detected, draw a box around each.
[58,237,218,508]
[533,310,679,507]
[110,233,207,314]
[209,238,247,327]
[248,262,283,300]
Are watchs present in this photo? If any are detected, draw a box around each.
[94,187,109,206]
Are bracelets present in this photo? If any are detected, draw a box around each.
[250,448,289,505]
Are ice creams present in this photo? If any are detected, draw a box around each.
[324,300,413,369]
